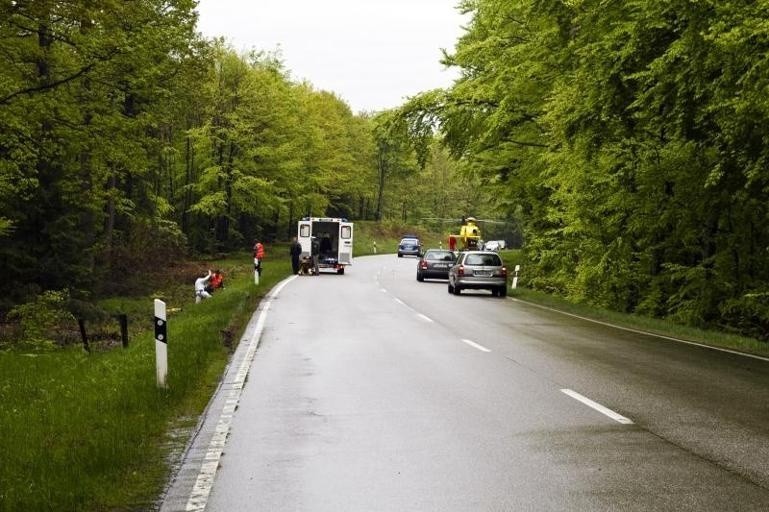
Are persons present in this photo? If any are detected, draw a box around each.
[289,237,302,275]
[204,270,226,296]
[310,235,320,276]
[194,268,213,305]
[253,239,265,277]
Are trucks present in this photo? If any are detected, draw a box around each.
[297,216,355,276]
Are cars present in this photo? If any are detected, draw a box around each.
[397,234,424,257]
[448,250,508,298]
[416,249,457,281]
